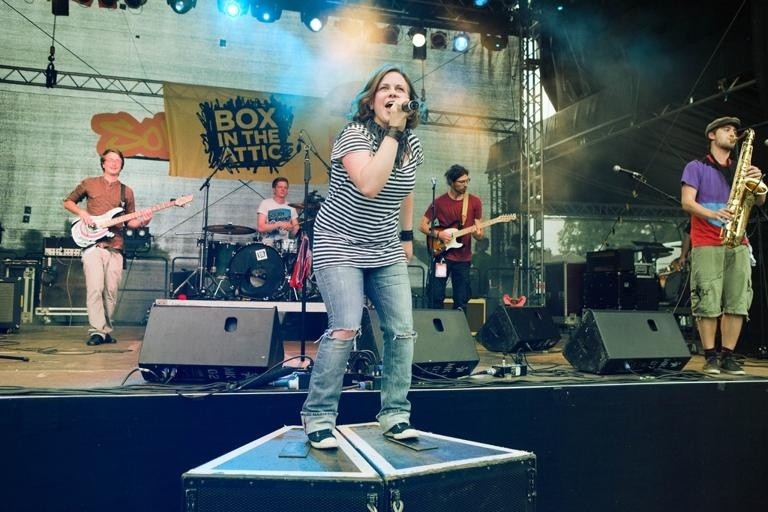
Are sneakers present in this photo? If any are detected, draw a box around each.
[87,335,103,346]
[102,333,117,344]
[719,357,745,375]
[309,429,340,449]
[384,423,420,439]
[702,356,720,374]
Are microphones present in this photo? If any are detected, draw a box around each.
[613,164,642,177]
[402,100,419,112]
[431,177,436,185]
[297,136,302,152]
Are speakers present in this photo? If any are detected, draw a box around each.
[138,300,284,381]
[474,304,561,353]
[335,421,536,512]
[182,426,385,512]
[563,309,691,373]
[355,305,479,378]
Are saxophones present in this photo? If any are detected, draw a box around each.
[720,128,768,248]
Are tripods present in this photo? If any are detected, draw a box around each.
[172,154,227,299]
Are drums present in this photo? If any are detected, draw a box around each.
[215,241,245,279]
[228,242,289,299]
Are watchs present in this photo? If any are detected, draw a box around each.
[140,222,146,228]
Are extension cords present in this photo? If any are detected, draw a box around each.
[294,370,353,388]
[364,376,382,390]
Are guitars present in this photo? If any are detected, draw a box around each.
[427,213,517,256]
[71,195,193,248]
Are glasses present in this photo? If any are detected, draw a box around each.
[455,178,470,184]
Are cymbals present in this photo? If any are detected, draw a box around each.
[202,224,255,235]
[288,203,320,208]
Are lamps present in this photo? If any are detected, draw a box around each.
[431,30,450,53]
[379,22,400,46]
[167,0,198,16]
[250,1,284,25]
[216,0,250,19]
[406,26,428,49]
[477,14,511,52]
[451,29,472,55]
[299,5,330,33]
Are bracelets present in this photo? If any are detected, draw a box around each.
[436,231,439,238]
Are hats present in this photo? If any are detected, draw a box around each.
[704,116,740,138]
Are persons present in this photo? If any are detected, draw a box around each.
[300,66,424,447]
[419,165,485,310]
[257,177,300,256]
[681,116,763,376]
[63,148,153,346]
